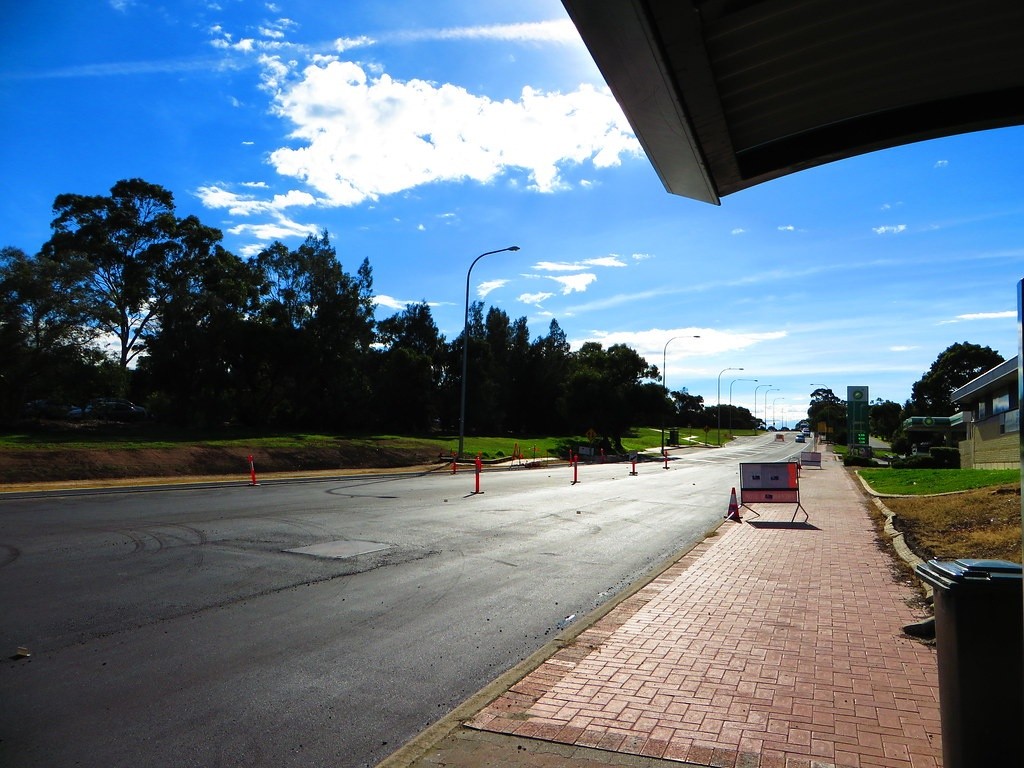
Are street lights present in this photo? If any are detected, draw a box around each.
[754,384,772,436]
[764,388,781,431]
[772,397,785,430]
[660,334,700,456]
[458,244,521,461]
[717,367,744,445]
[810,383,829,422]
[729,379,758,439]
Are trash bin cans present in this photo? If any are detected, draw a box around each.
[914,556,1024,768]
[670,430,679,445]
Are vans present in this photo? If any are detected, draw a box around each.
[800,424,808,431]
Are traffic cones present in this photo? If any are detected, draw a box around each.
[722,488,744,519]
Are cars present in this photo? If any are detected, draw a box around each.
[794,434,806,443]
[802,428,810,437]
[67,398,146,422]
